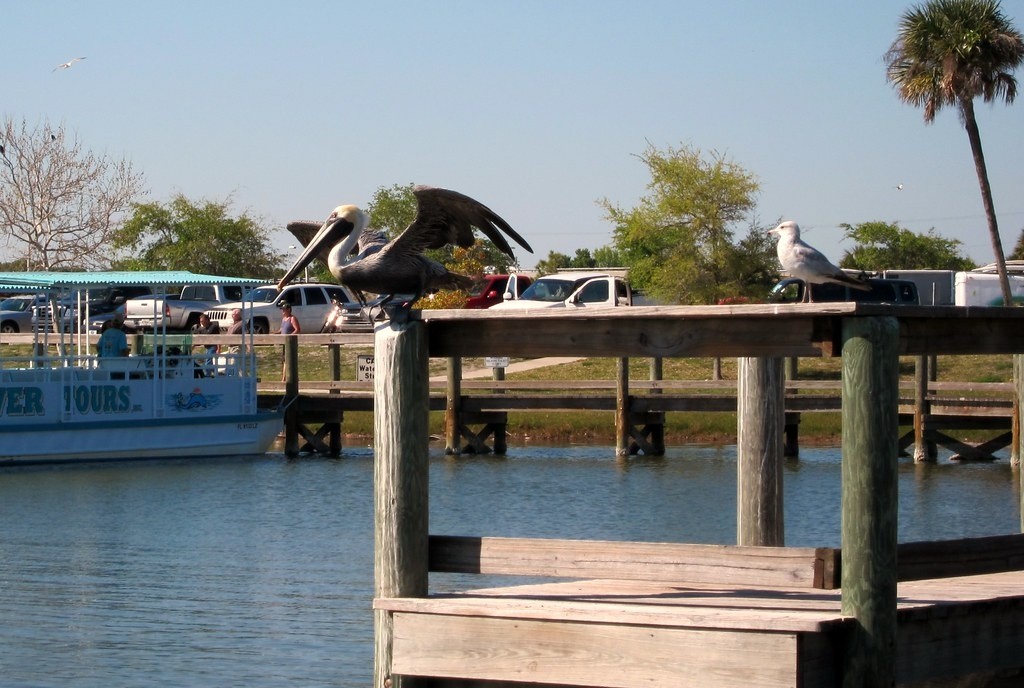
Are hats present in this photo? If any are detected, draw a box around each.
[113,313,127,324]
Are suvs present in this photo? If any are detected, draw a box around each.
[463,274,535,310]
[32,285,153,333]
[201,283,359,334]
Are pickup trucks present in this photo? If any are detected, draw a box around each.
[488,267,682,311]
[123,283,269,334]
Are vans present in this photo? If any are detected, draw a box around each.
[955,260,1024,307]
[839,269,954,308]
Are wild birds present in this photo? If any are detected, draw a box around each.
[766,220,872,304]
[277,186,534,325]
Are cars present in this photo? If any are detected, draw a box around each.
[339,293,416,330]
[0,296,60,333]
[82,294,181,334]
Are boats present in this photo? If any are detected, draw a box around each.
[0,271,286,463]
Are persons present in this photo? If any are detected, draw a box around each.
[95,316,129,357]
[273,304,300,381]
[225,308,250,368]
[192,314,222,378]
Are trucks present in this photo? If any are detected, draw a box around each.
[768,279,919,307]
